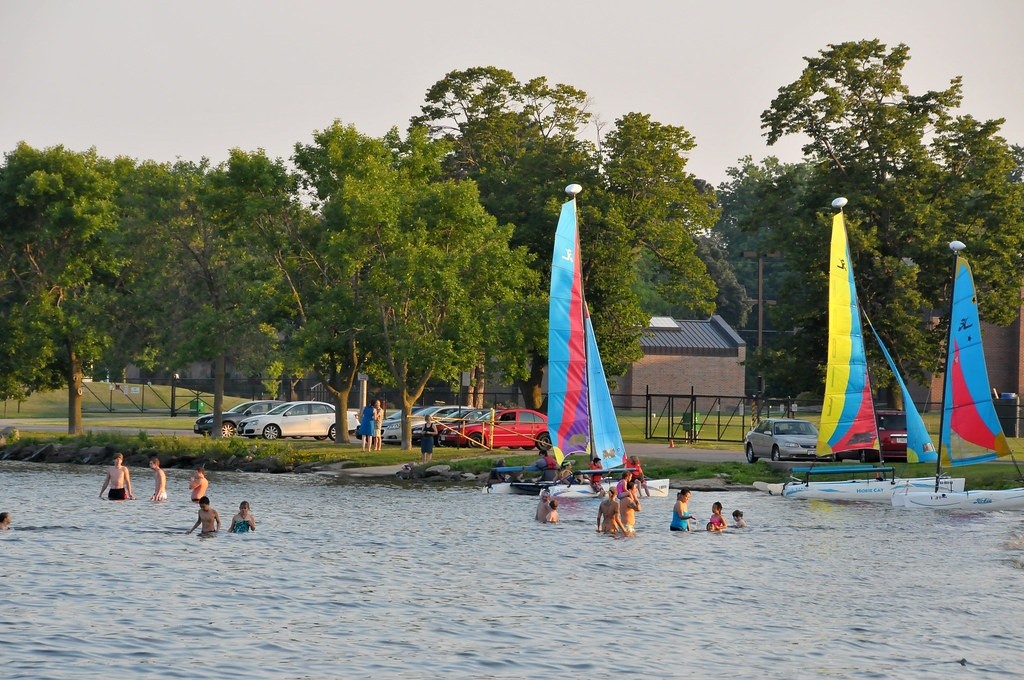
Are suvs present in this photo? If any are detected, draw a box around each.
[832,407,909,465]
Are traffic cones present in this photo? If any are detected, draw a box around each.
[668,439,675,448]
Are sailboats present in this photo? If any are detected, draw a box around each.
[765,197,966,503]
[889,240,1024,512]
[482,183,671,500]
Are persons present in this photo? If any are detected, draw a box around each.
[487,459,506,483]
[421,415,438,464]
[597,471,641,537]
[534,450,561,482]
[189,467,208,502]
[0,512,11,530]
[624,456,650,497]
[358,399,383,452]
[189,496,221,533]
[99,453,133,500]
[227,501,256,533]
[670,487,696,531]
[553,457,606,498]
[706,502,727,531]
[732,510,746,528]
[535,491,559,521]
[149,457,167,501]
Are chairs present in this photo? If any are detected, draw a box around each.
[299,407,305,414]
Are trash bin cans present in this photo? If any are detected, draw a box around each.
[190,400,205,417]
[683,413,701,431]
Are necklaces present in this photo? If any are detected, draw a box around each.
[241,515,248,519]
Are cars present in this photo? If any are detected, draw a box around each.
[191,401,305,440]
[235,400,362,442]
[353,403,489,449]
[442,409,553,453]
[743,420,836,463]
